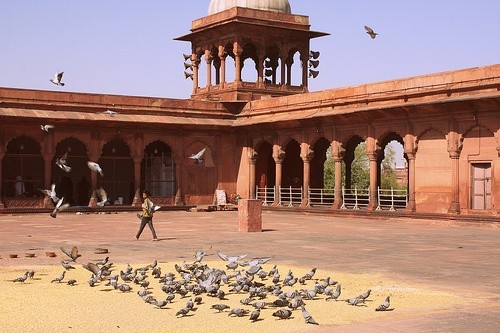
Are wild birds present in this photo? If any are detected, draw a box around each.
[55,152,72,173]
[10,247,391,326]
[37,122,55,134]
[49,71,65,86]
[188,148,207,165]
[87,161,104,177]
[364,26,379,40]
[40,184,70,219]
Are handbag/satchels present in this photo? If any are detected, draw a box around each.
[143,198,154,210]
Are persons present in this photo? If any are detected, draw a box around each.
[15,175,26,198]
[131,190,158,241]
